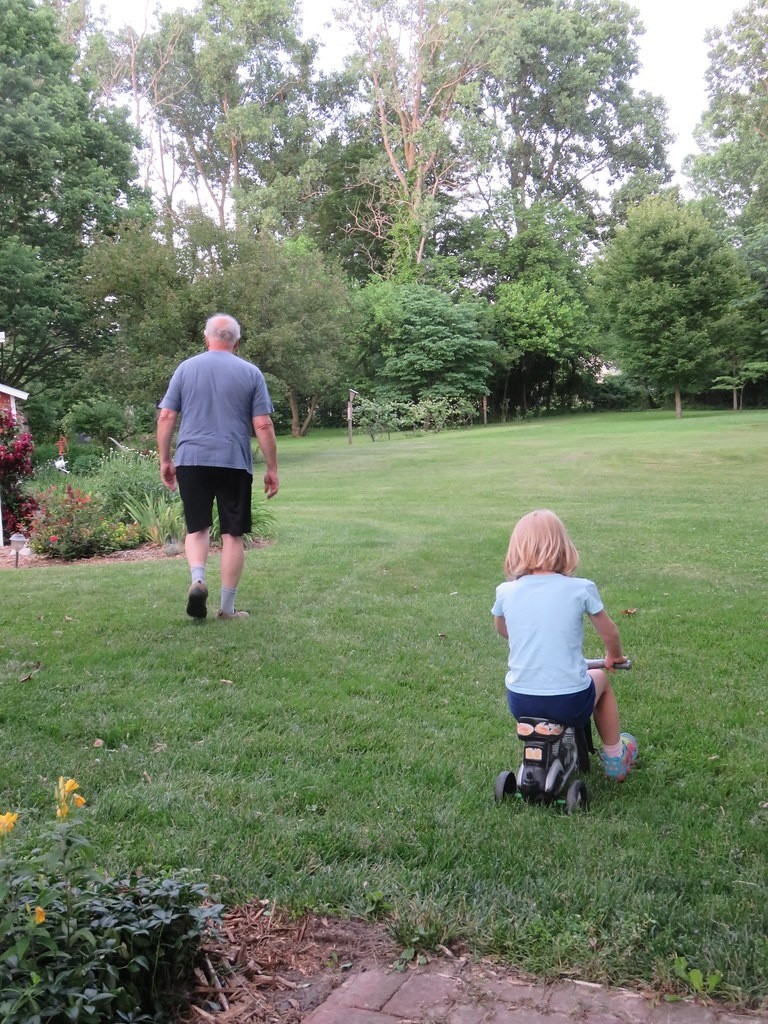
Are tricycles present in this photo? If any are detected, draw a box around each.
[494,659,633,817]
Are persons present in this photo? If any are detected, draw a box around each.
[488,509,638,783]
[156,312,279,621]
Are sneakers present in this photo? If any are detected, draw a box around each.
[601,732,637,780]
[217,610,249,621]
[187,582,206,619]
